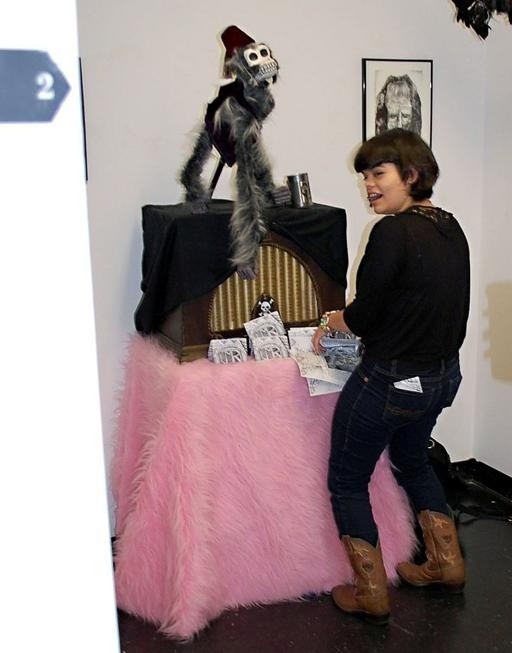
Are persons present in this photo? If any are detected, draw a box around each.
[311,129,473,624]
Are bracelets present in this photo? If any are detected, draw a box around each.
[319,310,341,340]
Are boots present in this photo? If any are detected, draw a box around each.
[331,535,390,623]
[396,510,465,593]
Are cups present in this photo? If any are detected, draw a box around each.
[284,171,313,209]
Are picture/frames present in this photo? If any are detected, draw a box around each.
[361,57,433,151]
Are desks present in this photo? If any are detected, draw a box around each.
[132,333,416,632]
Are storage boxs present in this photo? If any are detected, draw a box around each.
[136,197,351,367]
